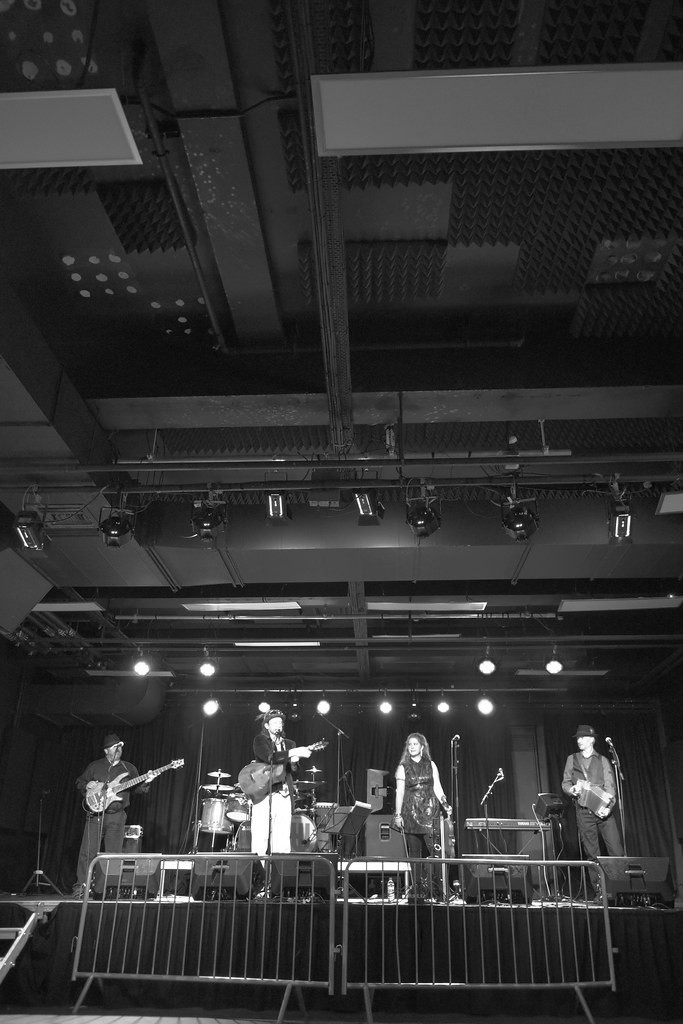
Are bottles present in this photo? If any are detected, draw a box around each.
[387,877,395,901]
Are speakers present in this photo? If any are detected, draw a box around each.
[93,852,162,899]
[461,853,534,905]
[122,838,142,853]
[270,853,338,900]
[365,768,408,862]
[596,855,674,908]
[191,851,265,901]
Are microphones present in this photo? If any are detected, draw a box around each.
[451,735,460,741]
[340,770,350,781]
[274,730,281,738]
[114,741,124,747]
[498,768,504,777]
[605,736,613,746]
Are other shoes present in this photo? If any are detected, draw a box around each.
[593,896,597,904]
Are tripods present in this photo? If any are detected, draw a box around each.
[320,806,373,903]
[20,790,64,896]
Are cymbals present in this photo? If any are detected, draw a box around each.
[207,772,232,778]
[201,784,235,791]
[292,780,326,790]
[305,769,322,772]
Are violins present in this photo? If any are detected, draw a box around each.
[441,795,456,858]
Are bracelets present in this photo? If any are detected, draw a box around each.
[395,813,401,819]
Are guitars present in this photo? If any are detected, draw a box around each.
[86,758,185,813]
[238,741,329,793]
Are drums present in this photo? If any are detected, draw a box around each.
[234,821,251,851]
[290,814,318,852]
[198,797,234,835]
[225,792,251,823]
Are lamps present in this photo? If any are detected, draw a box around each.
[190,492,228,540]
[356,487,384,527]
[546,653,564,674]
[199,656,217,677]
[134,650,152,678]
[401,480,442,542]
[498,464,540,543]
[265,491,287,519]
[606,483,633,543]
[477,651,497,675]
[17,487,48,549]
[95,491,135,550]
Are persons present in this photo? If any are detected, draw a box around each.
[562,725,624,902]
[72,734,158,900]
[394,733,452,902]
[251,710,312,868]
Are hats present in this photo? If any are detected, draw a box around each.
[262,710,287,728]
[572,725,599,737]
[100,733,124,749]
